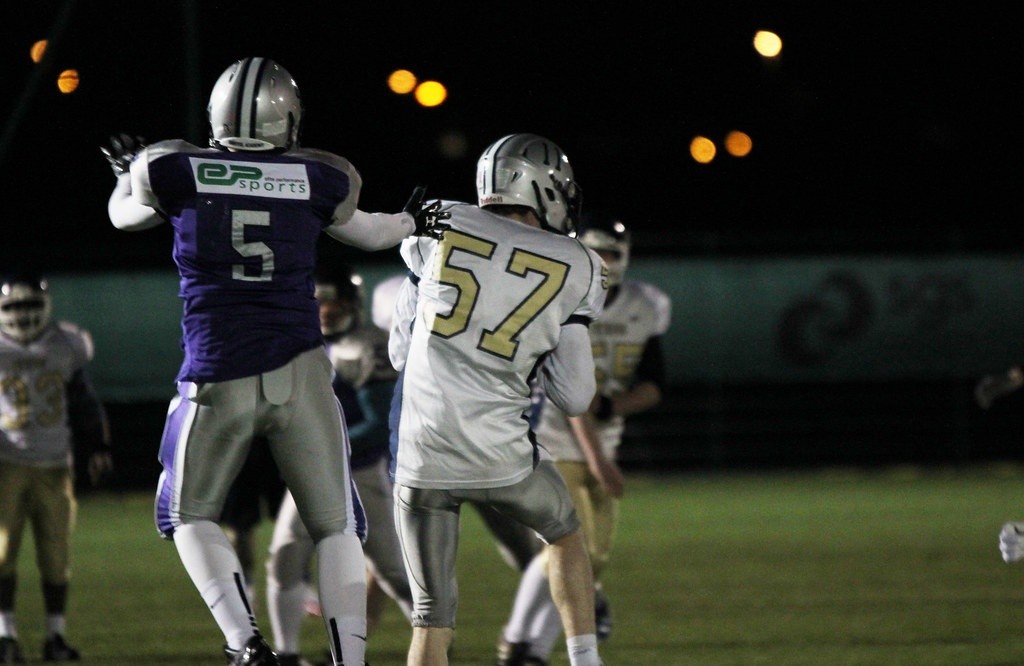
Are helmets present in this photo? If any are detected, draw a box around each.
[0,265,50,346]
[207,56,301,149]
[575,222,631,286]
[314,280,360,340]
[476,133,586,237]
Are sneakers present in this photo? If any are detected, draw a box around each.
[594,591,612,641]
[223,633,281,666]
[43,631,81,662]
[0,638,20,666]
[281,654,312,666]
[496,628,550,666]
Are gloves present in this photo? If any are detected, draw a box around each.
[403,186,452,240]
[100,133,147,177]
[85,451,114,485]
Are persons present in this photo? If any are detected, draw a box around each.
[228,219,678,665]
[101,57,452,666]
[0,276,113,665]
[386,133,613,666]
